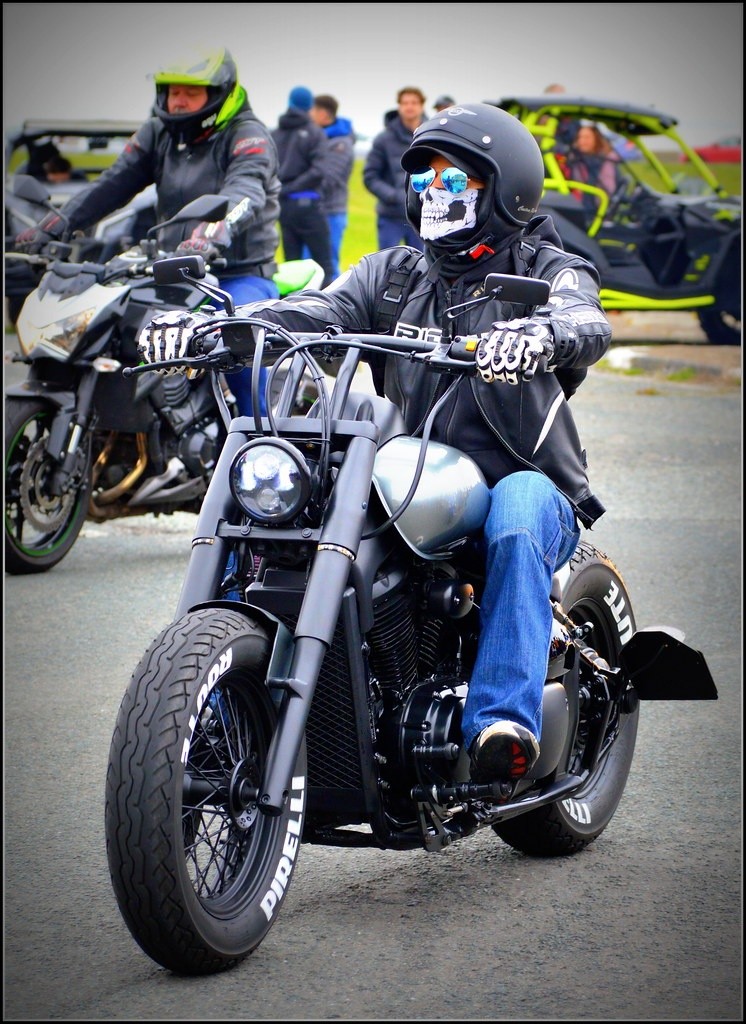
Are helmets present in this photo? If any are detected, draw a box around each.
[400,103,545,278]
[151,41,247,152]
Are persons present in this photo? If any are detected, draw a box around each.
[189,97,616,787]
[39,156,75,183]
[15,39,273,415]
[275,85,622,292]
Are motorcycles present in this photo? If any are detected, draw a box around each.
[101,253,719,978]
[6,166,333,580]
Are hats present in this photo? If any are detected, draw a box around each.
[288,86,314,111]
[434,96,453,106]
[314,96,338,110]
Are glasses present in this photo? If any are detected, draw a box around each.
[410,165,474,194]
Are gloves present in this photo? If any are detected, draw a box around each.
[476,318,555,385]
[15,211,65,256]
[136,310,222,378]
[175,221,231,261]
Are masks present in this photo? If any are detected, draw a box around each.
[419,187,479,241]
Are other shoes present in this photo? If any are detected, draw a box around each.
[200,705,219,729]
[468,720,540,782]
